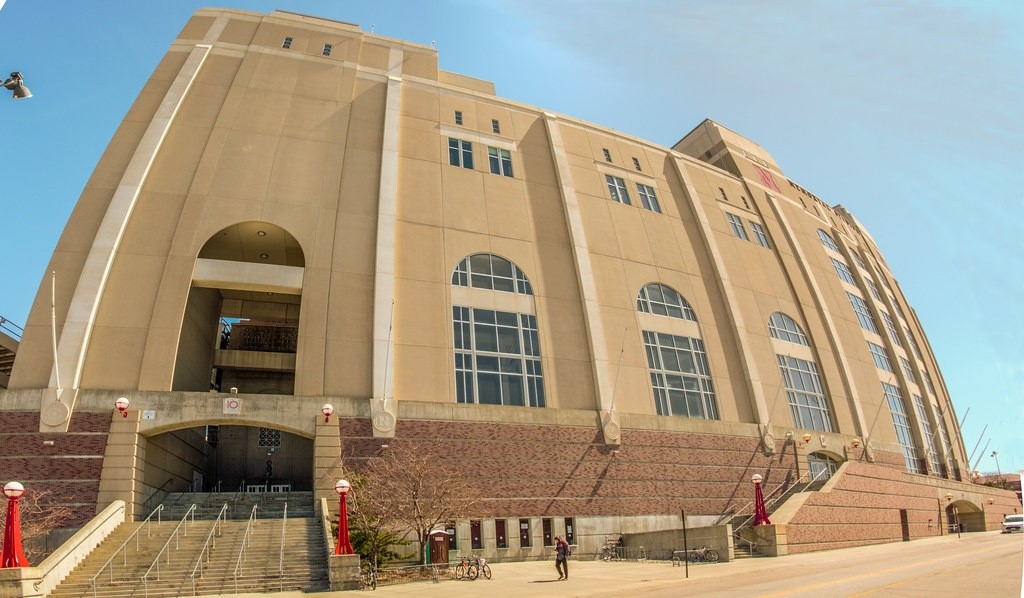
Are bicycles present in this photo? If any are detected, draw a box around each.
[471,554,492,579]
[455,556,479,580]
[361,562,377,589]
[688,545,720,563]
[603,545,622,563]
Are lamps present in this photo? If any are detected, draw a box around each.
[941,492,953,503]
[987,498,995,506]
[848,438,860,451]
[321,404,334,423]
[799,434,811,446]
[114,397,130,417]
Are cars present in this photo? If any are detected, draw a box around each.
[1000,514,1024,534]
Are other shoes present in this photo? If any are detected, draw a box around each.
[562,578,568,581]
[558,574,564,580]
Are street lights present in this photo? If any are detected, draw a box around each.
[751,473,771,525]
[334,479,354,555]
[1,481,28,566]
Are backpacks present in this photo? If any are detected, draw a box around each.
[562,540,571,556]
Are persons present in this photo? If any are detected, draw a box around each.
[553,536,570,581]
